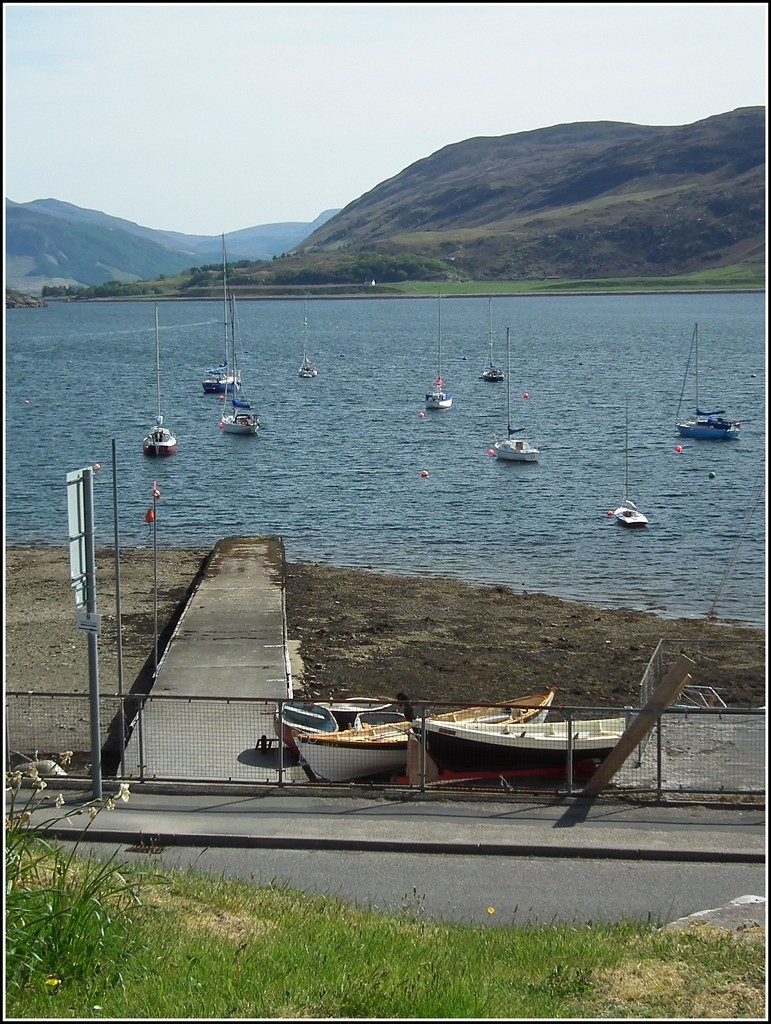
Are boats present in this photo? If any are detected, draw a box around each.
[291,689,554,782]
[314,695,397,729]
[274,697,340,750]
[411,717,626,781]
[354,712,406,730]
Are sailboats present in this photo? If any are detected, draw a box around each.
[673,323,741,441]
[613,388,649,527]
[425,289,454,410]
[202,233,242,392]
[141,301,176,456]
[482,296,505,381]
[490,324,542,464]
[220,292,261,434]
[297,300,317,378]
